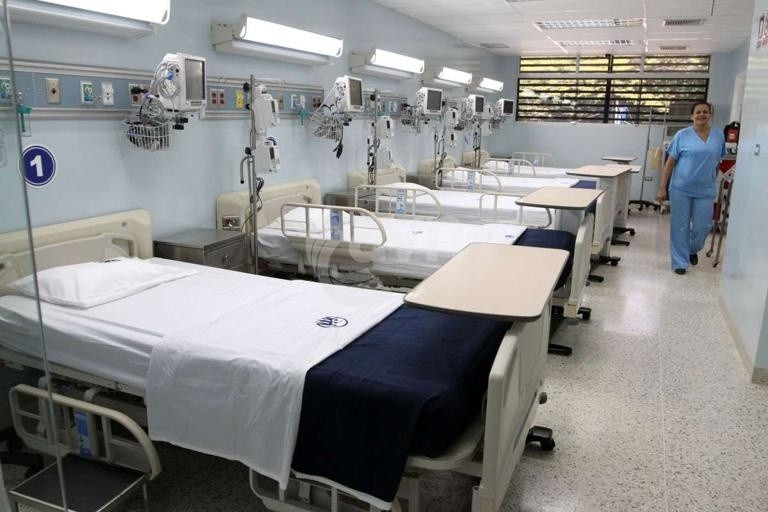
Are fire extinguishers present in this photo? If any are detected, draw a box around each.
[721,122,740,172]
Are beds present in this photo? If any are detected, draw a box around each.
[418,148,640,246]
[461,147,646,238]
[1,206,571,512]
[349,162,631,283]
[215,176,599,360]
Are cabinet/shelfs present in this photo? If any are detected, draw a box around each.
[326,187,376,211]
[152,224,250,271]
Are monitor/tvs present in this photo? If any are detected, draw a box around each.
[496,99,514,117]
[467,94,485,116]
[415,87,443,115]
[333,75,365,113]
[159,52,207,112]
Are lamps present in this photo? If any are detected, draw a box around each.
[2,0,173,41]
[353,46,432,84]
[209,9,350,71]
[467,76,508,96]
[423,56,473,93]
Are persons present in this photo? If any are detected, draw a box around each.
[655,101,728,274]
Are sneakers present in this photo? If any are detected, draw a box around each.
[675,268,686,274]
[690,253,698,265]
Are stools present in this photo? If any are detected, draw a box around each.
[7,454,154,512]
